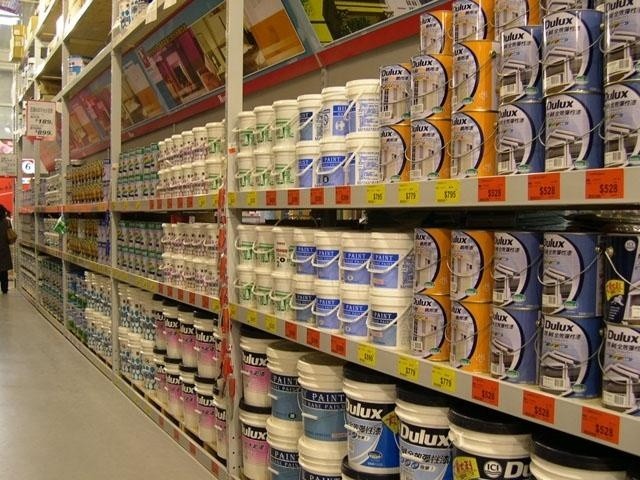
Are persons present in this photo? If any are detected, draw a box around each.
[0,203,17,294]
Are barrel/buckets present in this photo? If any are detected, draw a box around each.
[21,0,640,480]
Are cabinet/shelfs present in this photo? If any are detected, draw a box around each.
[9,0,640,480]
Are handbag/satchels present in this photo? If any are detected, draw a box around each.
[6,219,18,244]
